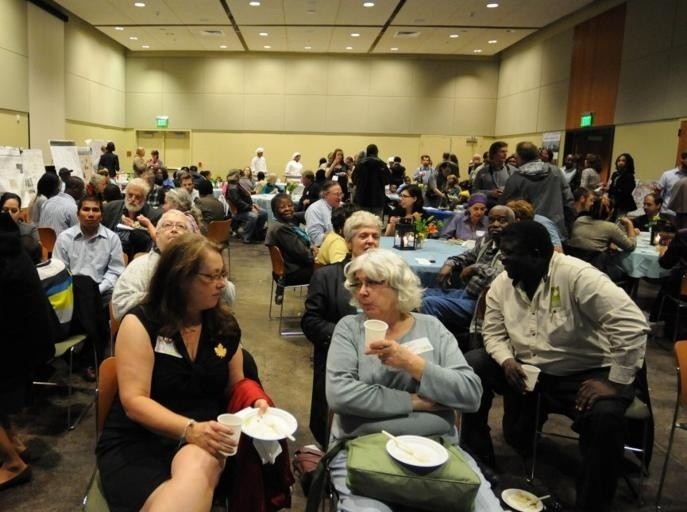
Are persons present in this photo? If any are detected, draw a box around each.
[1,133,281,488]
[269,141,687,451]
[458,220,650,512]
[319,245,506,511]
[94,235,274,512]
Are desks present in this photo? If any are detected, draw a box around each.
[612,230,677,298]
[377,235,474,287]
[252,193,304,211]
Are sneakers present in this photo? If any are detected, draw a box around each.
[240,233,252,243]
[19,448,40,464]
[0,465,31,491]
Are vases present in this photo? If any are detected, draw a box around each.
[415,238,426,249]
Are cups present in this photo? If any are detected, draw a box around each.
[362,319,388,349]
[217,413,243,458]
[520,363,540,393]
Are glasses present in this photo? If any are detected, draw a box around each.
[326,192,345,198]
[399,195,415,199]
[196,271,228,283]
[348,278,384,291]
[162,221,187,232]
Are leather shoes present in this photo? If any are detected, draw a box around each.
[79,365,97,381]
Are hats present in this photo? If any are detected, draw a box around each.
[256,148,264,154]
[59,168,72,176]
[292,152,301,160]
[468,192,488,206]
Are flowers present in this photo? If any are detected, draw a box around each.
[410,217,443,241]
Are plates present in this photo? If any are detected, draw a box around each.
[384,434,450,468]
[499,487,544,511]
[238,406,298,441]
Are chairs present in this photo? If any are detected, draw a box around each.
[653,339,686,508]
[84,356,133,511]
[53,276,100,431]
[205,220,233,280]
[655,270,687,350]
[266,245,313,337]
[564,243,639,301]
[37,228,56,261]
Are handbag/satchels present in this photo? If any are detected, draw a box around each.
[346,432,481,512]
[292,444,326,497]
